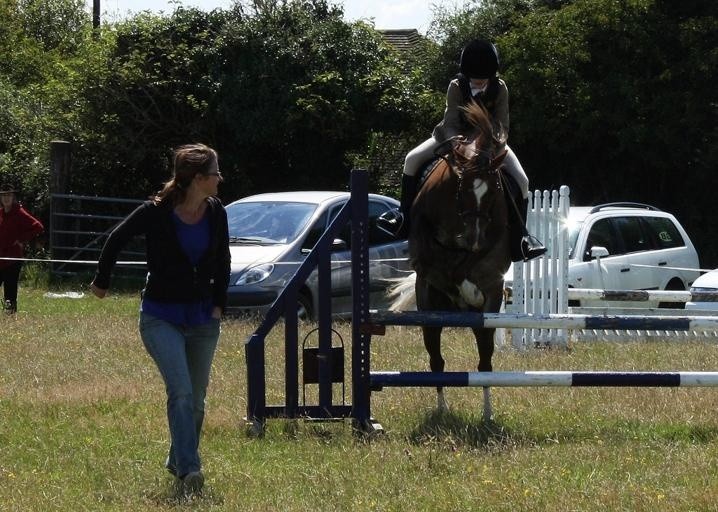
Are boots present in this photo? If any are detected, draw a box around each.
[376,174,417,241]
[508,198,547,262]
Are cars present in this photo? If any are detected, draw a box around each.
[683,265,717,310]
[139,189,412,324]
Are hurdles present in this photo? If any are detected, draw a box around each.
[247,169,718,441]
[497,184,717,349]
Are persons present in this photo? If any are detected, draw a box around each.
[91,144,232,494]
[0,183,43,315]
[377,38,547,263]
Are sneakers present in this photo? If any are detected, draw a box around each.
[166,463,205,504]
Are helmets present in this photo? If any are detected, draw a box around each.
[460,41,498,79]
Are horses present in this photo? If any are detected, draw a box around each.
[377,98,512,422]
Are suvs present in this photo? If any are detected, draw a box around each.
[500,198,703,307]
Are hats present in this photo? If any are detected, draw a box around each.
[0,184,19,193]
